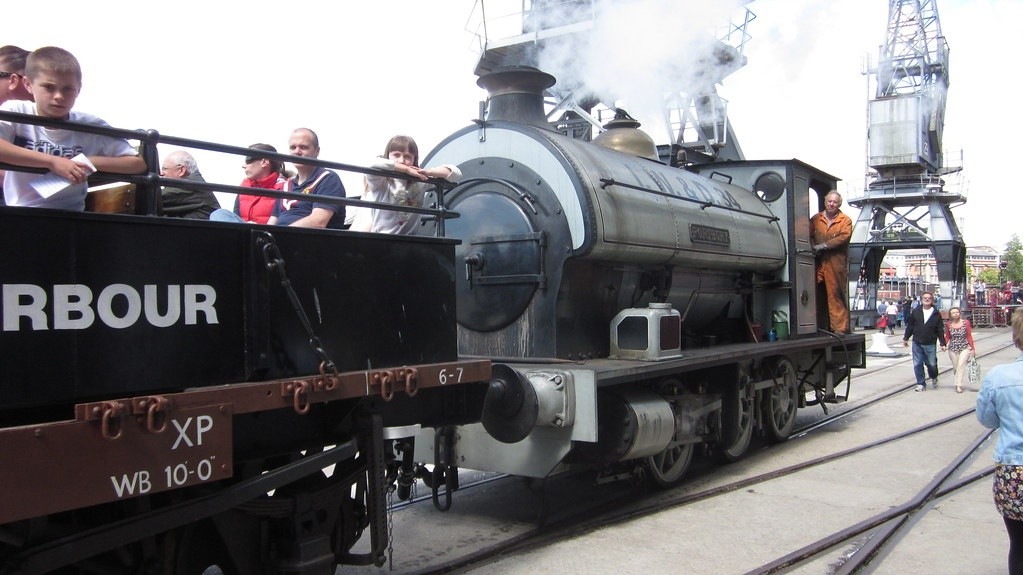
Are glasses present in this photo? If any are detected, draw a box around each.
[245,155,262,165]
[922,296,933,300]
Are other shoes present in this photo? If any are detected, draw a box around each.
[915,384,928,392]
[956,386,963,393]
[930,378,939,390]
[891,330,894,335]
[834,331,846,338]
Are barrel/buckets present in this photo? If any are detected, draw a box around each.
[747,318,763,343]
[773,322,788,341]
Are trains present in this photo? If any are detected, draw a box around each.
[0,63,866,575]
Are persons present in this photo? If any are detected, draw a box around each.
[975,279,986,306]
[210,128,346,229]
[809,190,852,338]
[878,293,941,334]
[976,306,1023,575]
[0,45,147,211]
[1012,281,1023,305]
[903,292,947,392]
[940,307,976,393]
[366,135,463,236]
[160,150,221,220]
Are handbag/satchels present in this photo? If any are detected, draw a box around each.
[968,356,980,383]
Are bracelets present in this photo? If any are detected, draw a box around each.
[823,243,827,250]
